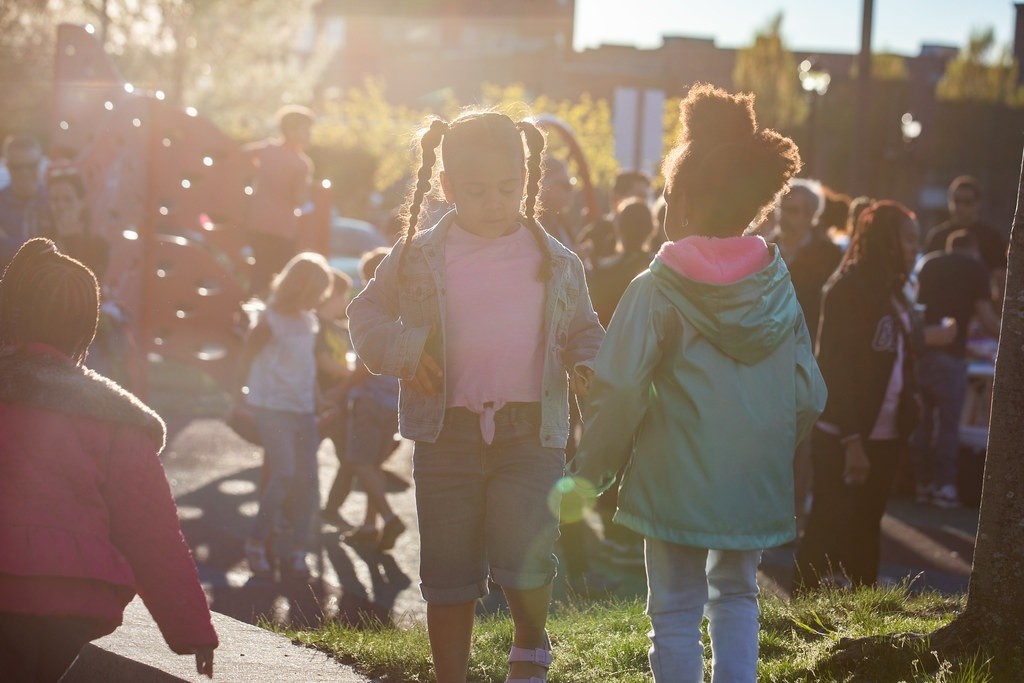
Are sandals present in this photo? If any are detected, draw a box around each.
[504,628,553,683]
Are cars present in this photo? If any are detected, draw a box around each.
[795,230,1002,463]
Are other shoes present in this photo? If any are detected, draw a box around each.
[320,508,354,531]
[913,475,959,509]
[378,516,404,549]
[596,537,643,563]
[340,526,378,546]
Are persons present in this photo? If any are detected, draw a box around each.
[1,103,1008,628]
[0,238,218,683]
[580,84,829,682]
[347,111,606,683]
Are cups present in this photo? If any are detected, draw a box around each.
[941,317,957,327]
